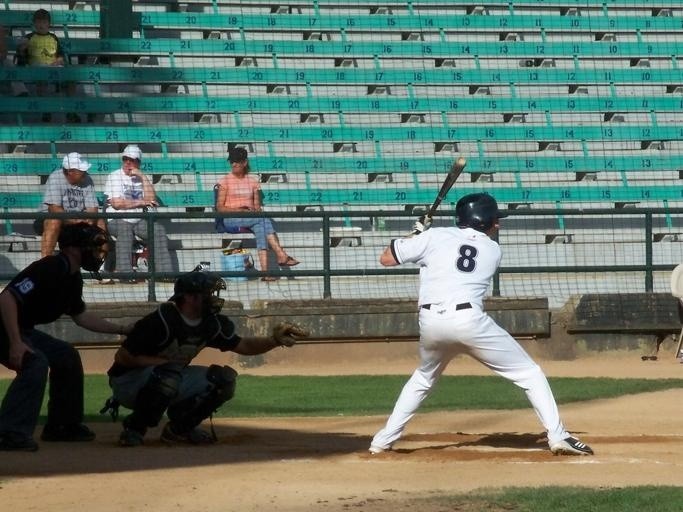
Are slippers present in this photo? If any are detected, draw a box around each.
[278,254,300,266]
[260,275,279,282]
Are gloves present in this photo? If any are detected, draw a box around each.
[413,214,432,237]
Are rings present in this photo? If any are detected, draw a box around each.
[132,170,133,173]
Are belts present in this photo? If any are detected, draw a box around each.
[420,302,474,311]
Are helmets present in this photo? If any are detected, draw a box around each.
[60,221,110,273]
[455,193,511,229]
[174,265,227,317]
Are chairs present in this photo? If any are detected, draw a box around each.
[670,264,683,357]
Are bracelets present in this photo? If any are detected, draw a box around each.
[116,323,124,335]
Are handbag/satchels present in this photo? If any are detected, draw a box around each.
[220,252,251,282]
[133,243,148,283]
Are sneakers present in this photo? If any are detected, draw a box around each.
[0,433,39,452]
[118,426,144,446]
[161,425,214,445]
[39,422,96,441]
[548,436,594,457]
[369,441,394,454]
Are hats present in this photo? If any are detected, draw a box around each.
[227,148,247,161]
[62,152,92,172]
[120,144,142,160]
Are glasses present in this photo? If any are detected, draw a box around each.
[121,156,138,163]
[229,159,243,164]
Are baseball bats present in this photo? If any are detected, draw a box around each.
[426,157,466,227]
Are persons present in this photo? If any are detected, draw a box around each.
[364,190,594,458]
[32,151,115,285]
[105,270,311,447]
[0,221,135,455]
[213,147,300,284]
[103,142,185,282]
[0,27,29,125]
[14,8,83,124]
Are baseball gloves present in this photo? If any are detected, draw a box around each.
[272,321,310,348]
